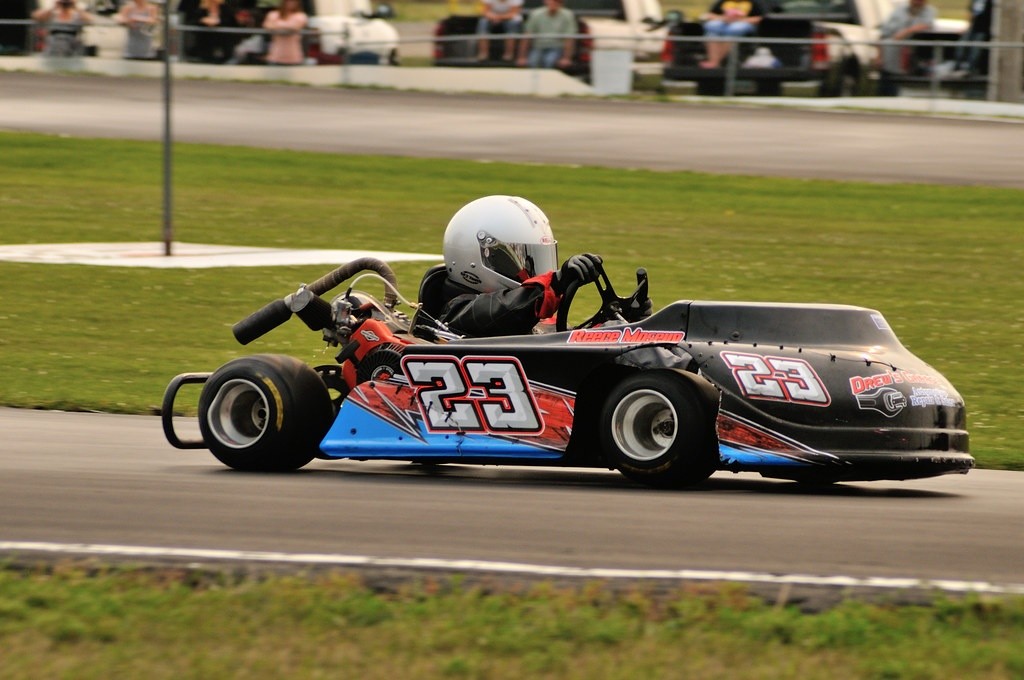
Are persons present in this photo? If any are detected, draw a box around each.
[34,0,990,97]
[440,195,653,338]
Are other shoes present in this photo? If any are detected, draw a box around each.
[931,59,974,80]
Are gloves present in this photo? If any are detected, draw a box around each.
[549,252,605,299]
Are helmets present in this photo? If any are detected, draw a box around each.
[441,193,558,295]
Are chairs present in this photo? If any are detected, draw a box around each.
[408,263,450,343]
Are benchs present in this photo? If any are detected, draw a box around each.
[183,4,280,65]
[879,29,990,89]
[662,17,843,94]
[431,13,593,86]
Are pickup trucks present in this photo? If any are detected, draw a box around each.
[665,0,896,96]
[432,0,671,72]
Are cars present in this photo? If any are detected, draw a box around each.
[179,0,402,69]
